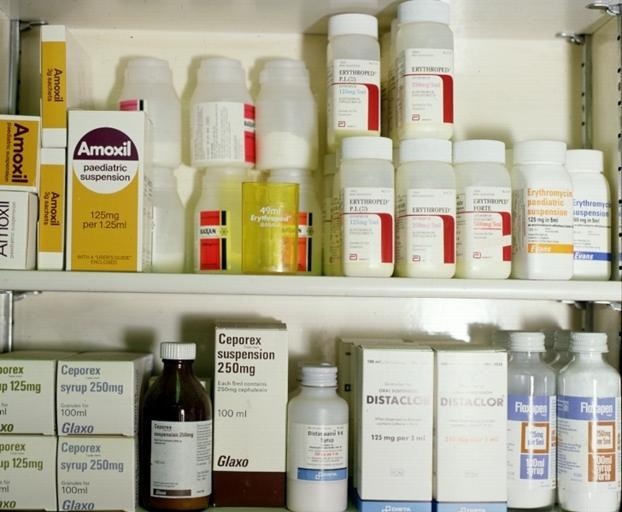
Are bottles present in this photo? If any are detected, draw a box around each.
[397,0,453,140]
[389,18,398,139]
[140,342,212,510]
[262,171,323,276]
[395,139,456,277]
[452,139,514,278]
[507,332,558,511]
[568,150,612,279]
[194,167,257,273]
[254,61,320,170]
[511,141,574,280]
[556,333,621,512]
[550,331,578,374]
[190,57,256,168]
[506,148,513,175]
[115,57,181,168]
[327,14,381,148]
[146,172,185,272]
[331,172,344,275]
[322,154,332,274]
[286,364,350,511]
[379,32,389,139]
[288,363,334,410]
[337,137,394,276]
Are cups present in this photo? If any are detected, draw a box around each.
[240,180,301,275]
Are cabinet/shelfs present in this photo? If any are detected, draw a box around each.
[0,0,621,512]
